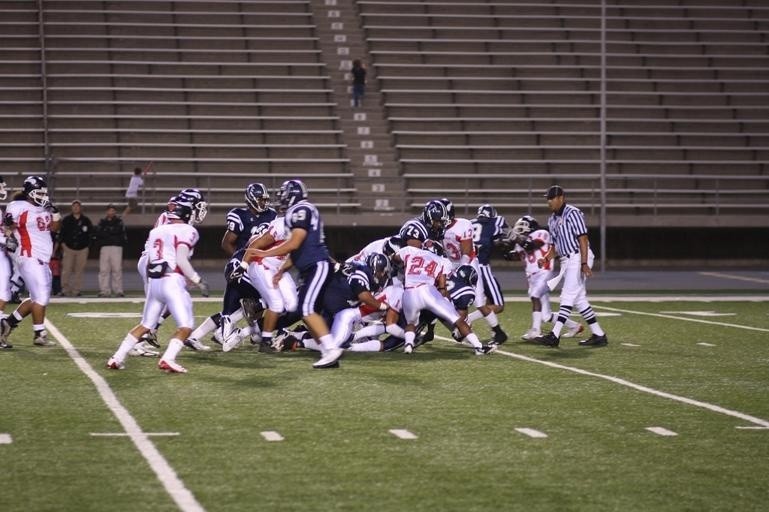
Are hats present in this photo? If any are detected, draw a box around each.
[544,185,564,196]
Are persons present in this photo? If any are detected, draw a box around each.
[123,168,147,214]
[106,179,606,373]
[350,59,368,107]
[0,174,127,348]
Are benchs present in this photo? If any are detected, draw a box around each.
[1,0,769,229]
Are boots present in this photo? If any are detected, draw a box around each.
[32,330,55,346]
[1,314,18,348]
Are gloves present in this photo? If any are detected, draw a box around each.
[2,214,14,228]
[507,230,523,244]
[199,281,211,298]
[43,201,62,221]
[4,238,19,253]
[230,261,248,284]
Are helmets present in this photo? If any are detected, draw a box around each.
[23,175,50,207]
[457,265,478,288]
[513,215,538,234]
[0,176,8,200]
[476,204,498,218]
[168,188,208,223]
[245,179,309,214]
[367,198,455,279]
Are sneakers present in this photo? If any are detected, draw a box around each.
[404,343,414,355]
[452,317,507,356]
[535,331,560,347]
[313,347,345,368]
[521,330,544,340]
[239,298,257,327]
[158,359,186,372]
[562,323,584,338]
[106,356,126,370]
[129,314,314,357]
[579,333,607,345]
